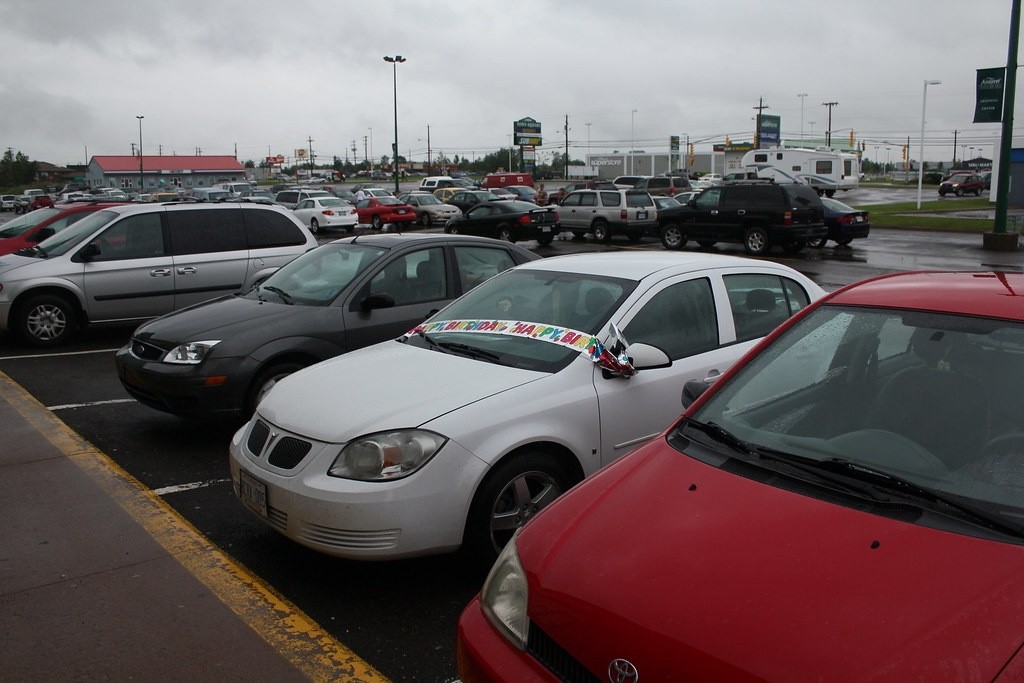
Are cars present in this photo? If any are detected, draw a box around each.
[113,233,547,434]
[458,271,1024,683]
[444,196,567,248]
[229,249,915,584]
[554,172,720,207]
[818,196,871,247]
[0,169,539,237]
[937,170,993,197]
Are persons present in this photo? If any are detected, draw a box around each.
[355,188,365,201]
[534,184,548,205]
[557,187,569,204]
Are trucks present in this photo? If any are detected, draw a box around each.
[740,146,860,197]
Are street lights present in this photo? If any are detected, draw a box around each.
[873,146,984,168]
[916,78,942,209]
[798,94,816,139]
[585,108,637,174]
[136,115,145,192]
[384,55,407,194]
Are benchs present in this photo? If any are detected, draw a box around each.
[660,283,781,341]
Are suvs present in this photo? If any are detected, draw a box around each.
[543,188,659,245]
[0,198,160,257]
[0,199,317,353]
[656,181,828,258]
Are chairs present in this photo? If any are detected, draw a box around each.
[865,365,988,471]
[376,256,441,301]
[571,287,617,331]
